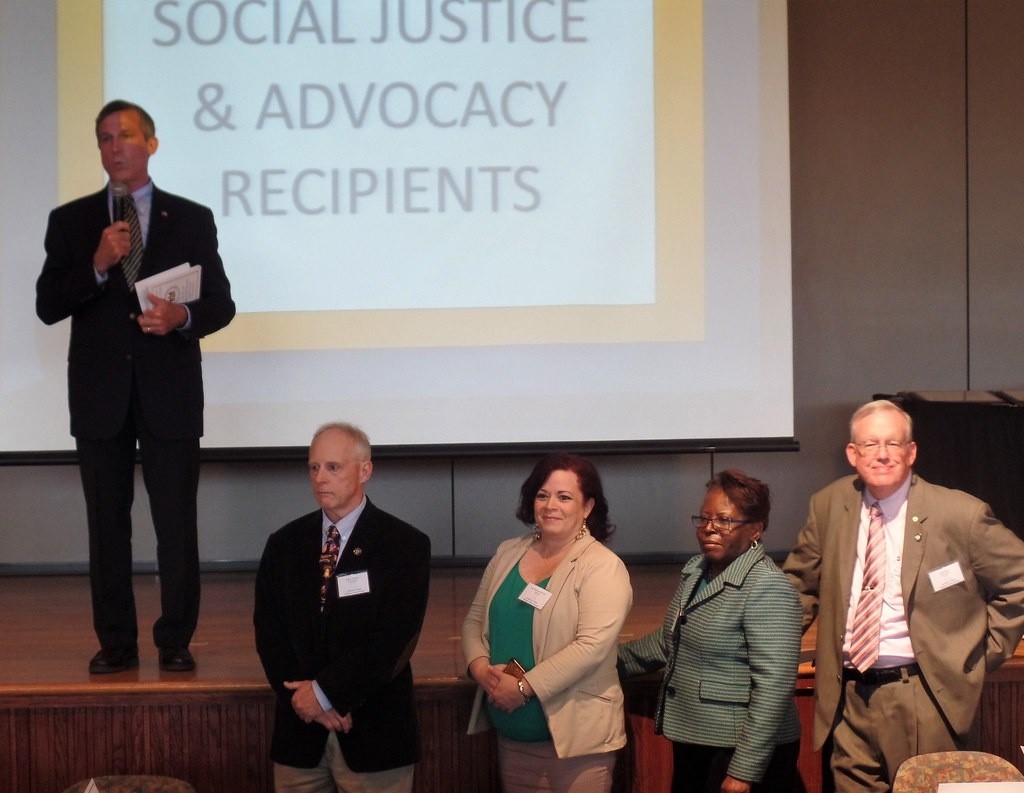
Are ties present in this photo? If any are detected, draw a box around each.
[850,504,887,673]
[112,195,145,292]
[318,526,341,611]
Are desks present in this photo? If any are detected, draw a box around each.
[875,393,1023,542]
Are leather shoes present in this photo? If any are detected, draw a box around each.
[89,647,139,675]
[158,635,195,671]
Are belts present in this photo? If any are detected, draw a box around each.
[842,662,919,683]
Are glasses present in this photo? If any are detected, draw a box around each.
[693,515,756,530]
[852,440,911,449]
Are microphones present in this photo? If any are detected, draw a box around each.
[110,182,128,232]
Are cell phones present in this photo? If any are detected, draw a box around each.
[503,658,525,682]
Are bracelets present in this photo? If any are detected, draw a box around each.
[517,679,529,702]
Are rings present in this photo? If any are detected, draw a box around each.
[147,327,150,332]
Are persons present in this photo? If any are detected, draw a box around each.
[617,468,805,792]
[782,399,1023,792]
[254,422,436,793]
[459,451,633,793]
[37,98,236,677]
